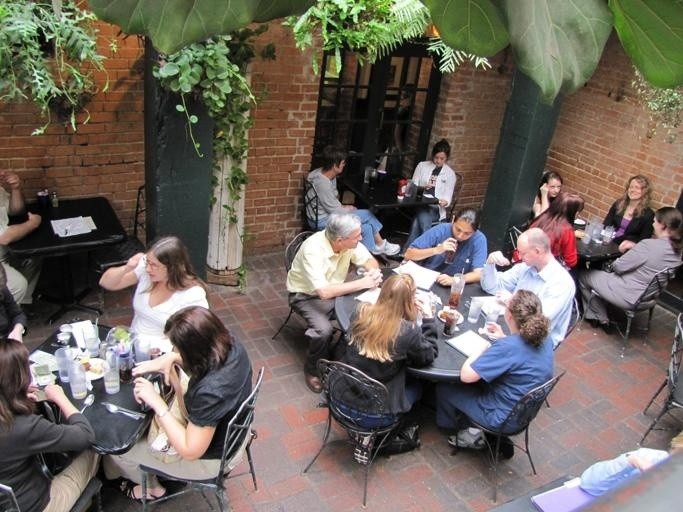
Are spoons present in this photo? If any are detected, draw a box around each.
[104,404,141,421]
[78,393,95,414]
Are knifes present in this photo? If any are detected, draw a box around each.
[100,402,146,419]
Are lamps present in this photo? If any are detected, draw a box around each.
[44,84,95,116]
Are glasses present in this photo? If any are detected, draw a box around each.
[141,257,174,269]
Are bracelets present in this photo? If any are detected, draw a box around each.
[154,407,171,418]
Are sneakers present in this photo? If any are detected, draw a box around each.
[346,430,375,466]
[446,429,487,452]
[20,303,38,319]
[370,238,406,268]
[591,318,611,331]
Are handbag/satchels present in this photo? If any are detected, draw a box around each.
[139,372,173,412]
[30,450,72,482]
[378,421,421,457]
[146,362,193,465]
[28,388,64,425]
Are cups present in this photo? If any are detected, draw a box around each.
[54,325,162,400]
[363,165,425,202]
[441,273,501,338]
[582,216,614,246]
[444,240,456,265]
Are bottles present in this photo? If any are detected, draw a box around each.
[36,190,60,208]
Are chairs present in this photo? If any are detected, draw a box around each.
[611,267,675,344]
[137,365,266,512]
[271,230,337,363]
[301,172,320,233]
[552,296,580,354]
[637,310,683,444]
[449,366,570,503]
[396,171,464,227]
[0,474,105,512]
[506,227,523,252]
[301,357,405,508]
[91,184,162,310]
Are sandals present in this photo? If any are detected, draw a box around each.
[119,479,170,505]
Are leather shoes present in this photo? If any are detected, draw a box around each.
[303,364,322,394]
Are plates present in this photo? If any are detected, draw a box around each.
[437,309,465,324]
[573,218,586,225]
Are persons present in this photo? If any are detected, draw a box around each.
[404,207,489,286]
[588,176,654,269]
[102,307,254,504]
[0,168,43,344]
[285,209,383,393]
[579,207,682,327]
[510,192,584,317]
[0,338,102,512]
[304,147,401,257]
[331,272,439,458]
[402,136,455,252]
[530,169,562,221]
[434,289,555,451]
[480,227,577,351]
[98,234,210,353]
